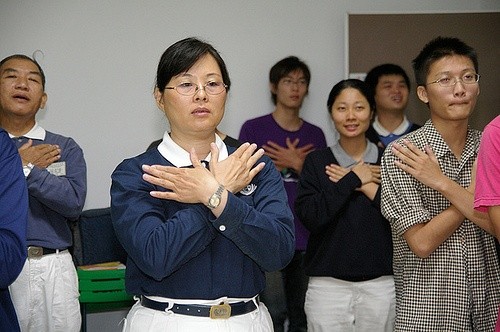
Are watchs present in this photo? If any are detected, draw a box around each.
[23,162,34,177]
[206,184,224,210]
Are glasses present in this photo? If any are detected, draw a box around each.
[164,79,228,96]
[281,77,308,87]
[424,72,480,87]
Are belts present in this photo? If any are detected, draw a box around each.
[27,244,67,258]
[139,294,260,319]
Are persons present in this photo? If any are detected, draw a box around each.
[110,36,294,332]
[238,36,500,332]
[0,54,87,332]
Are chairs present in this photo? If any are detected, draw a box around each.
[77,207,137,332]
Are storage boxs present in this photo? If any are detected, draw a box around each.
[79,262,130,301]
